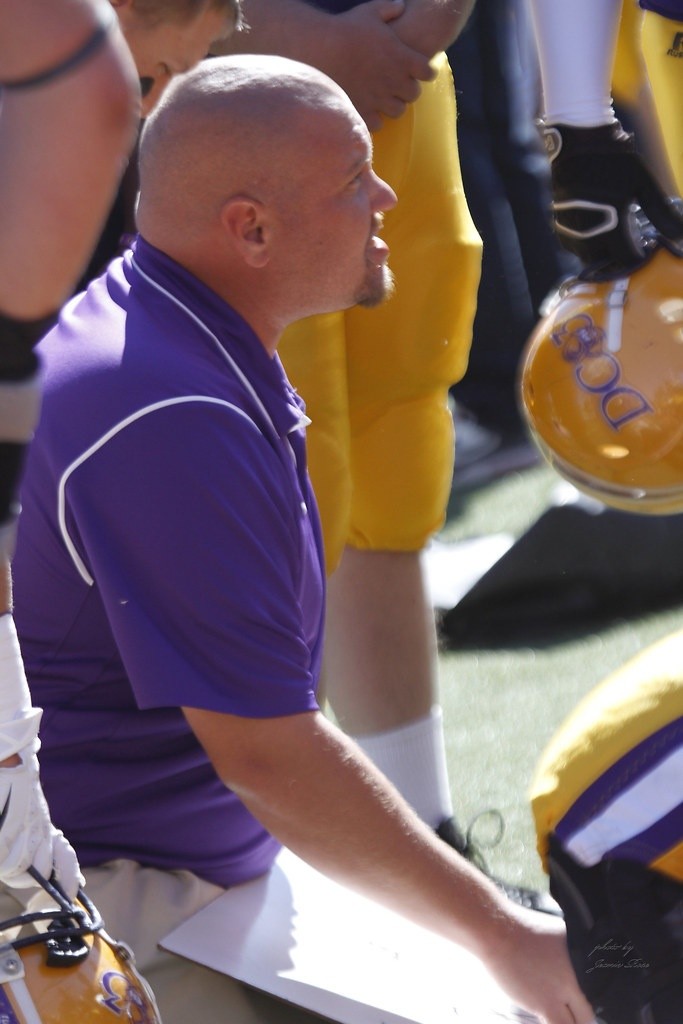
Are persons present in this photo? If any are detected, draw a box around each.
[0,0,683,1024]
[11,53,594,1024]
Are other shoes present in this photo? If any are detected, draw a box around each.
[434,814,564,919]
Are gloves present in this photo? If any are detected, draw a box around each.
[0,762,86,902]
[537,116,683,283]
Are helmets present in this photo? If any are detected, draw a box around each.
[520,249,683,515]
